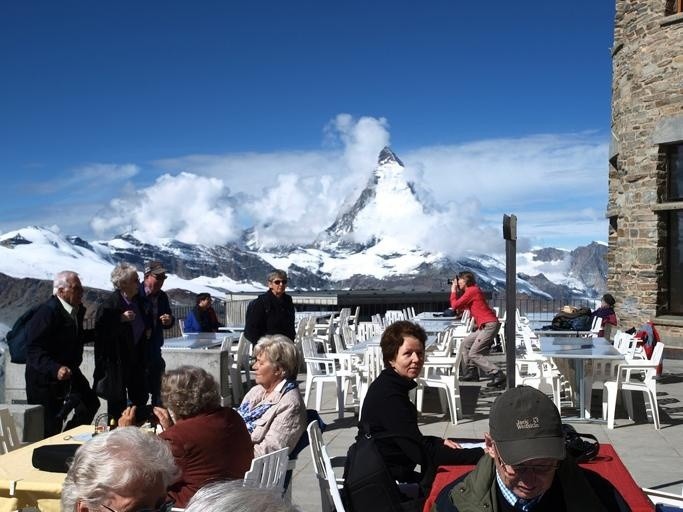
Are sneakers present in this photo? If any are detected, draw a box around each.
[489,345,506,353]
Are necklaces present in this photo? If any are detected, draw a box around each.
[261,378,282,401]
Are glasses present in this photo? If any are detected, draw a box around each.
[269,279,287,285]
[101,493,177,512]
[493,444,561,475]
[151,273,167,280]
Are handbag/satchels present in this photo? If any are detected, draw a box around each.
[91,350,124,403]
[560,423,600,465]
[32,444,82,473]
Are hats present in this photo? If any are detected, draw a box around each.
[561,305,572,314]
[143,261,168,275]
[488,385,566,466]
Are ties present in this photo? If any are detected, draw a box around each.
[518,493,542,512]
[72,309,77,336]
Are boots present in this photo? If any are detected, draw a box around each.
[487,369,506,387]
[459,366,480,381]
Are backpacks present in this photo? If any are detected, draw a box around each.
[6,299,59,364]
[342,428,435,512]
[551,307,592,331]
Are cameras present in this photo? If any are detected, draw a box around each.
[133,404,154,424]
[563,424,597,461]
[56,392,80,422]
[448,275,458,284]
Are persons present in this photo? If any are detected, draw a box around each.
[591,294,617,337]
[242,269,296,380]
[118,365,254,512]
[183,292,231,346]
[131,261,175,408]
[91,261,143,427]
[429,383,632,511]
[448,271,506,387]
[55,424,182,512]
[5,270,100,438]
[337,320,489,511]
[230,332,306,478]
